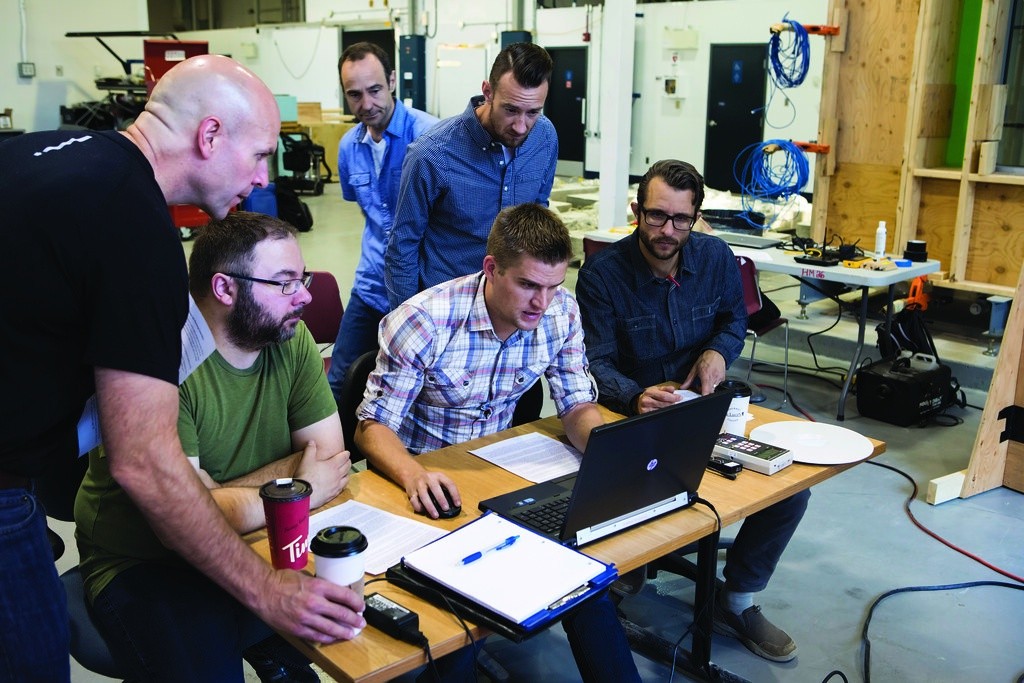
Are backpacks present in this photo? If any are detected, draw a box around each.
[274,175,314,233]
[876,302,940,363]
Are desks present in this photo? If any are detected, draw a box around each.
[241,379,888,681]
[302,123,358,182]
[586,225,939,421]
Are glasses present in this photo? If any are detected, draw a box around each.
[636,202,697,230]
[211,272,314,295]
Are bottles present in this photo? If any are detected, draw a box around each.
[875,221,887,257]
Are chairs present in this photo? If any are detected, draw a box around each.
[279,130,330,193]
[341,351,544,463]
[584,238,790,405]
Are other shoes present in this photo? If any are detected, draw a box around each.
[610,565,647,597]
[712,590,799,662]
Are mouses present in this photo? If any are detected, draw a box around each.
[417,486,461,520]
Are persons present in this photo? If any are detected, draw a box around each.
[1,53,368,683]
[351,204,642,683]
[383,42,560,452]
[73,212,351,683]
[577,159,812,663]
[329,41,441,462]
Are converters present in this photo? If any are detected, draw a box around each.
[362,592,419,641]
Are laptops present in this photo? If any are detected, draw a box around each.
[717,233,782,249]
[478,389,734,549]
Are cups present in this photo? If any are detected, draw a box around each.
[259,478,313,571]
[310,526,368,636]
[714,380,752,438]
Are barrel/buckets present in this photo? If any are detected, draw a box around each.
[897,351,940,374]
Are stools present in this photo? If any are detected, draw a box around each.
[59,564,273,679]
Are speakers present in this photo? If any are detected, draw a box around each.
[501,30,531,49]
